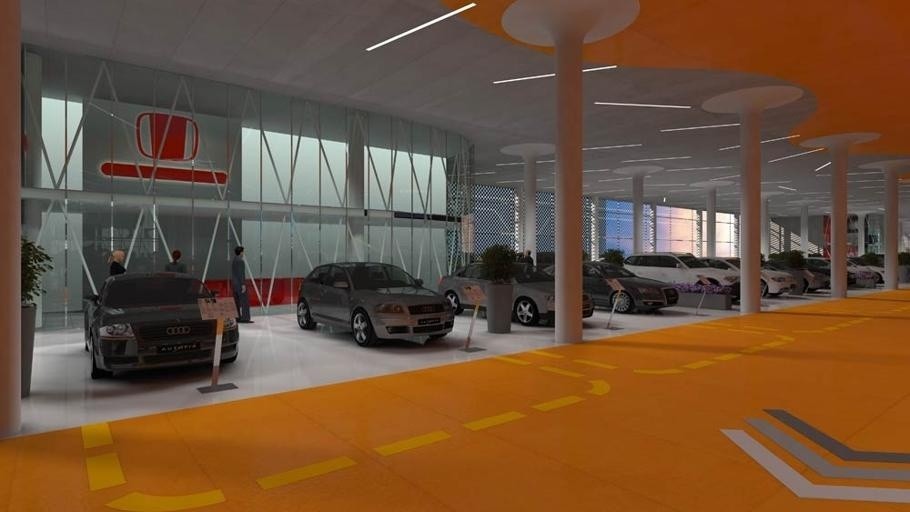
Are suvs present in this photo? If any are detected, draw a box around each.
[292,260,454,353]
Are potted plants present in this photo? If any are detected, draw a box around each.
[783,249,804,294]
[21,233,55,398]
[479,243,525,333]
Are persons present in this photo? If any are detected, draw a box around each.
[108,248,126,275]
[165,249,187,274]
[523,250,533,264]
[230,245,255,324]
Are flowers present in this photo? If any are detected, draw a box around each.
[672,282,732,295]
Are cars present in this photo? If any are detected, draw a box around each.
[437,261,598,330]
[540,260,679,314]
[622,252,909,304]
[82,263,242,383]
